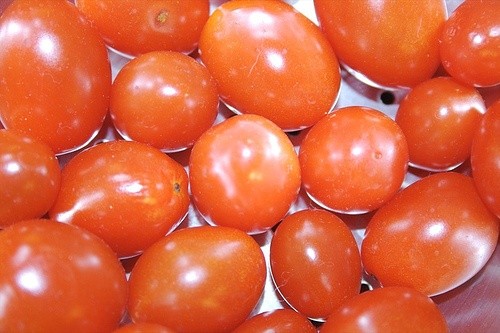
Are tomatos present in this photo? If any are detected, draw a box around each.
[0,0,500,333]
